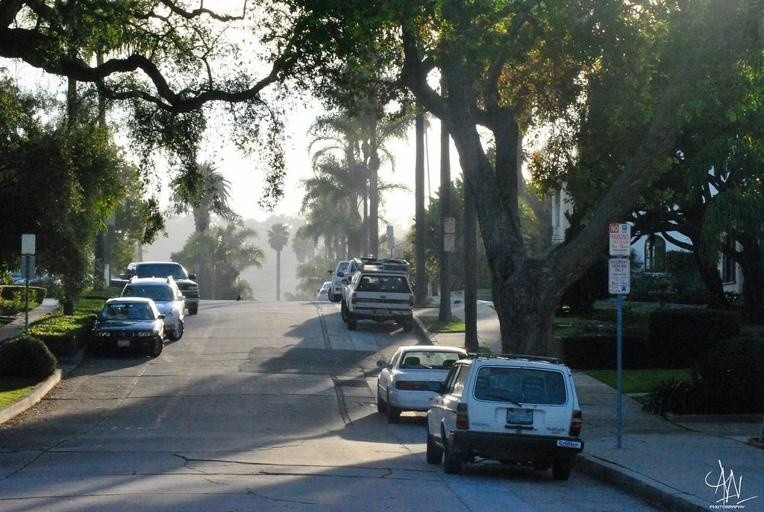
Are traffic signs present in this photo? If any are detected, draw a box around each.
[608,258,630,295]
[609,223,630,257]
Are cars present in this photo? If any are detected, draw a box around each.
[89,297,165,357]
[316,257,414,331]
[120,275,186,342]
[377,345,469,424]
[120,261,200,314]
[0,272,95,297]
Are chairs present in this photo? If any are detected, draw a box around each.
[522,377,545,392]
[443,360,456,365]
[405,357,420,365]
[477,376,490,386]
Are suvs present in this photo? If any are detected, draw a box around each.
[426,352,584,481]
